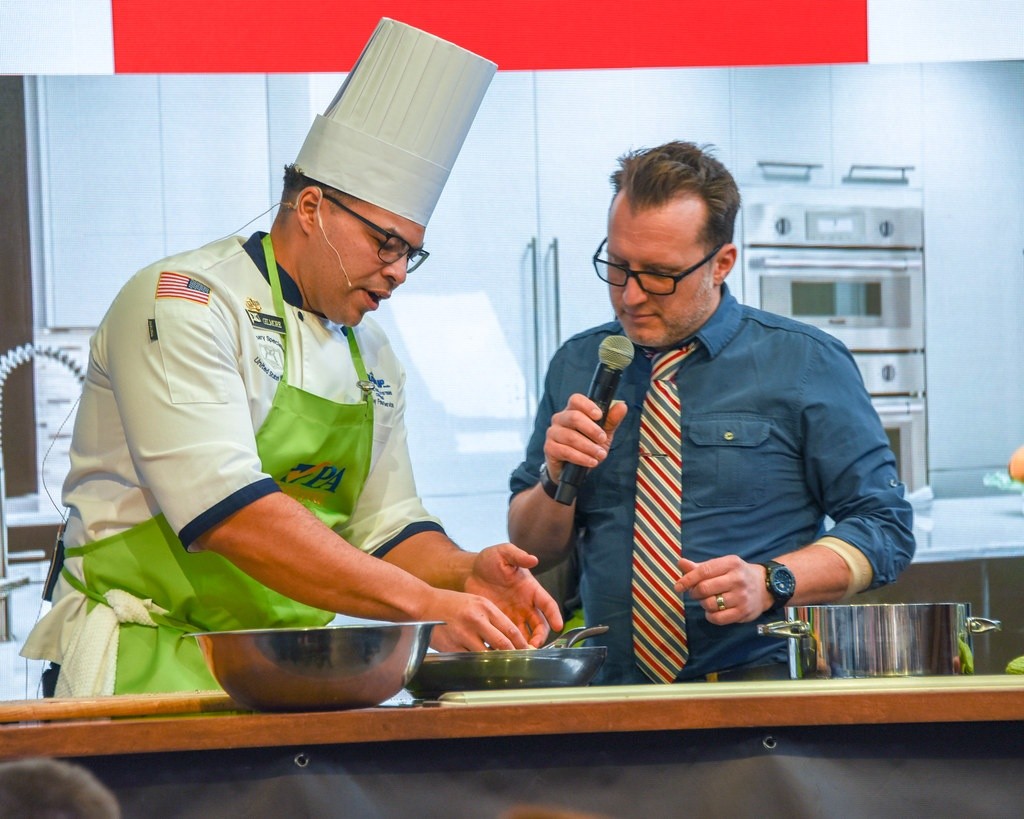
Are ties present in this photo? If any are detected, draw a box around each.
[632,341,700,684]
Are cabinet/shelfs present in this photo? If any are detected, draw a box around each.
[35,73,272,328]
[270,67,735,501]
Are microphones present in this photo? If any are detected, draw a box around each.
[318,216,353,288]
[554,334,635,507]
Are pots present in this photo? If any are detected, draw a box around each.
[762,598,1004,686]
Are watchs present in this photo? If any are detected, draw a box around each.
[756,558,799,611]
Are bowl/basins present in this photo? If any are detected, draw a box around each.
[180,614,448,709]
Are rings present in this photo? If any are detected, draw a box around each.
[713,592,729,614]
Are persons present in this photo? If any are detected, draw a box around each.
[27,114,565,706]
[505,138,917,685]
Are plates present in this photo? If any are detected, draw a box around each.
[400,642,609,698]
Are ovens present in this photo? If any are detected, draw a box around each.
[732,188,929,509]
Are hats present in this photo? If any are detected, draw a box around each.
[290,15,499,228]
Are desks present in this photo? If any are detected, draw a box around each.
[0,672,1023,818]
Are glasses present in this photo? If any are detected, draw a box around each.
[590,236,725,297]
[323,192,429,275]
